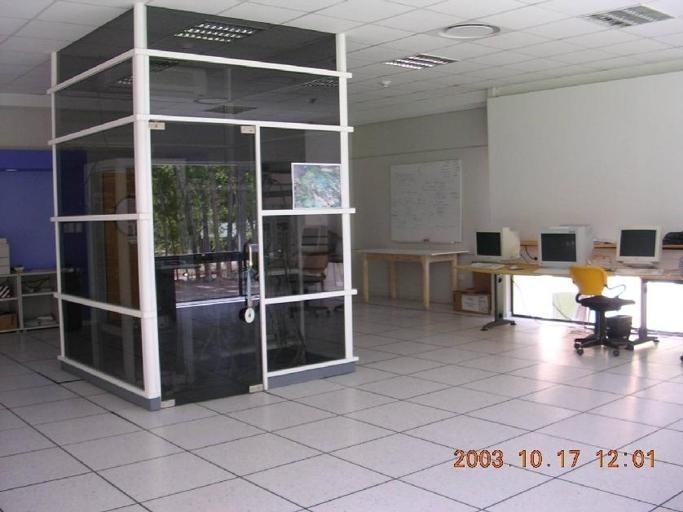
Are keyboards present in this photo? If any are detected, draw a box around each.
[470,261,504,271]
[533,265,573,275]
[616,265,662,276]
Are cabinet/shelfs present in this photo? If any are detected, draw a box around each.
[0,271,60,333]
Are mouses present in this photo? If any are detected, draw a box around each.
[508,263,525,270]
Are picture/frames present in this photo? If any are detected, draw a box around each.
[114,196,137,236]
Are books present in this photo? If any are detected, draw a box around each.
[0,282,11,298]
[24,314,57,328]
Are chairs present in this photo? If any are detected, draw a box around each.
[568,265,634,357]
[288,226,330,308]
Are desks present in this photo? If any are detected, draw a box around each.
[351,248,470,311]
[455,260,683,331]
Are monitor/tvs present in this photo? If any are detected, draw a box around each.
[617,225,663,266]
[538,224,598,267]
[474,228,522,262]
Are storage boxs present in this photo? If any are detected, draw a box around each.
[453,288,491,314]
[0,312,17,329]
[0,238,11,275]
